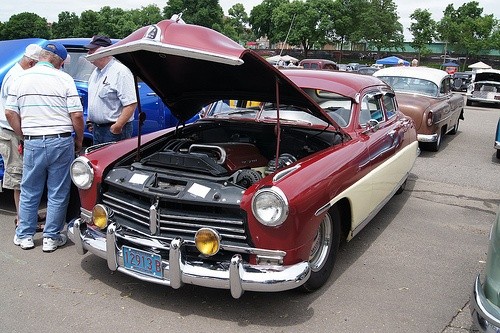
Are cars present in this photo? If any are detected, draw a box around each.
[345,62,359,70]
[67,14,420,299]
[443,66,500,109]
[0,38,231,194]
[362,67,464,153]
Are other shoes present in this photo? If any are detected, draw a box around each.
[14,212,46,224]
[15,224,44,232]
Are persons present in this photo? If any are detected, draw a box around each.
[412,59,417,67]
[84,36,136,146]
[398,60,404,66]
[279,60,294,66]
[0,41,84,251]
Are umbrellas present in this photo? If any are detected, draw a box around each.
[444,62,459,67]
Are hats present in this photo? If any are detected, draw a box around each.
[42,41,67,68]
[84,36,112,49]
[25,44,41,61]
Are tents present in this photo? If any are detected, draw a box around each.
[468,62,491,69]
[267,54,299,63]
[376,56,410,67]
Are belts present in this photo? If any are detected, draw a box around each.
[25,132,71,139]
[95,123,115,127]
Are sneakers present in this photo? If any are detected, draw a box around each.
[14,235,34,249]
[43,234,67,251]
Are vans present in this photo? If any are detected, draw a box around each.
[298,58,338,70]
